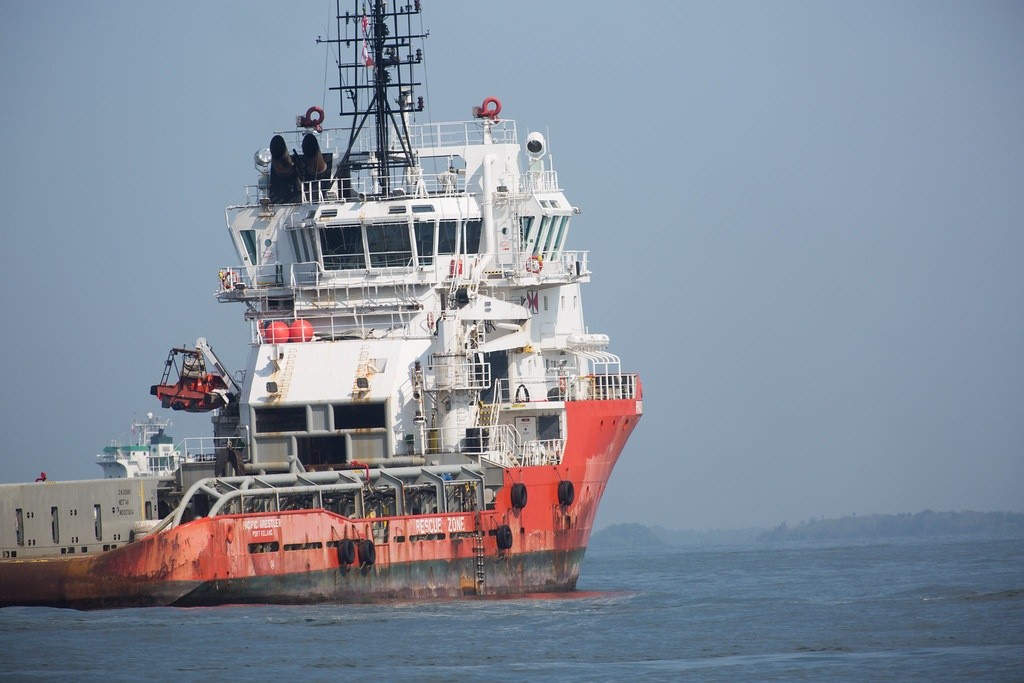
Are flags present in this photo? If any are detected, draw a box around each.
[361,40,374,67]
[361,16,368,36]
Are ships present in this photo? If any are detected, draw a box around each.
[0,0,644,611]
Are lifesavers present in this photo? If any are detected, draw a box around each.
[512,480,528,510]
[558,373,568,391]
[339,538,356,566]
[359,538,377,565]
[426,312,433,327]
[559,481,574,505]
[527,255,543,274]
[496,524,512,548]
[221,271,238,288]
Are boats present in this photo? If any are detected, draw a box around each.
[150,375,229,414]
